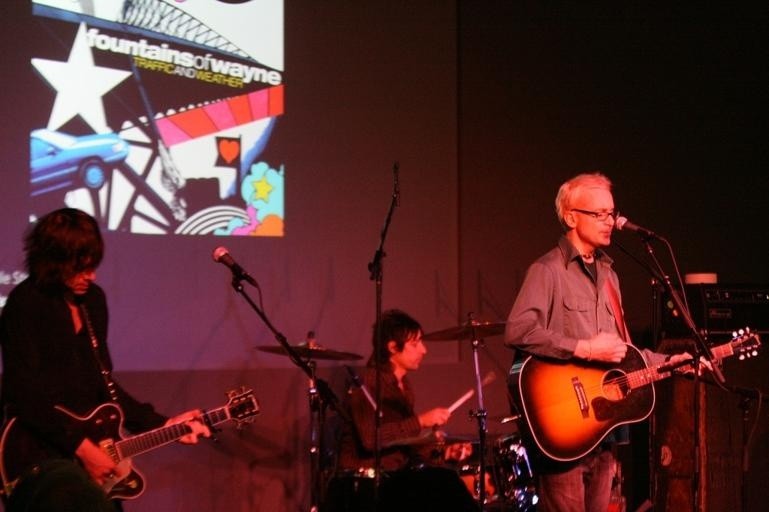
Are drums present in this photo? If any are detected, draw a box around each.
[454,447,535,503]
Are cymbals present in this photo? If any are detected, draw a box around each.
[419,322,507,341]
[257,344,365,362]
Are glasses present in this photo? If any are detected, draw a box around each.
[571,209,621,222]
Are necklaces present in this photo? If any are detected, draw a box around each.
[581,250,593,259]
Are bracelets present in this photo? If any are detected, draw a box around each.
[665,354,676,361]
[588,339,592,362]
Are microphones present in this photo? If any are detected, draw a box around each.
[213,247,258,288]
[616,216,668,244]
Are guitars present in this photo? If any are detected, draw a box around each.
[510,324,761,468]
[2,387,259,510]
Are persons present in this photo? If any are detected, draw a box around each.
[0,207,211,512]
[6,458,116,512]
[323,309,477,512]
[502,173,705,512]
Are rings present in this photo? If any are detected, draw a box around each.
[109,474,114,479]
